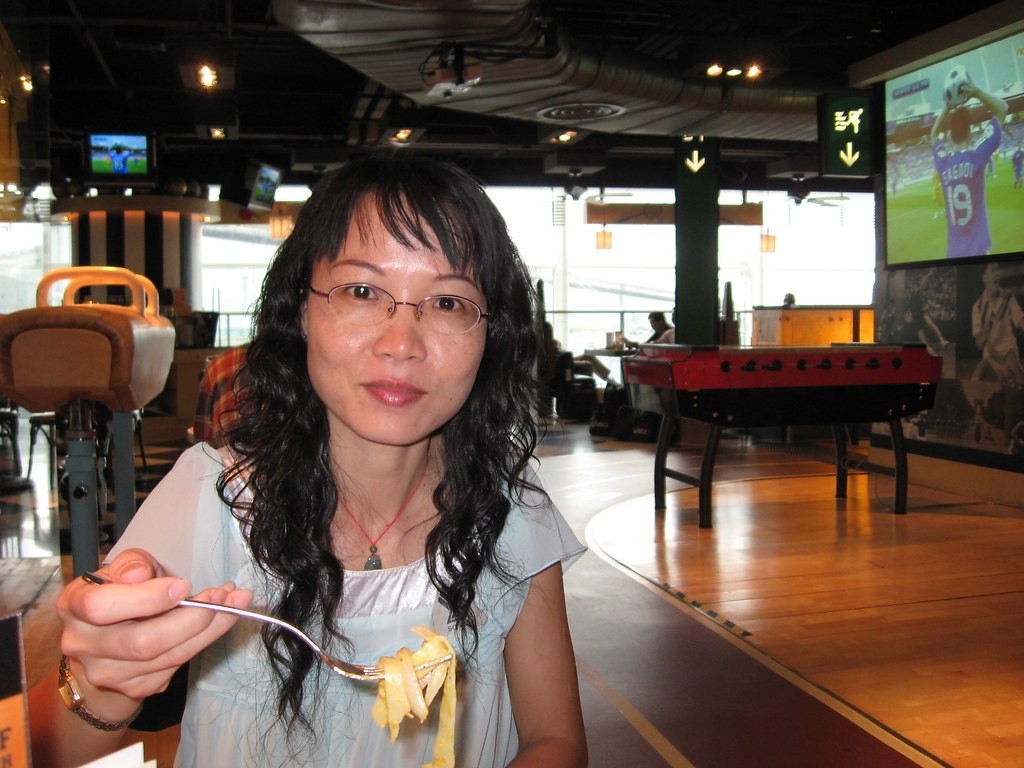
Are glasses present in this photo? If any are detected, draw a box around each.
[309,282,493,336]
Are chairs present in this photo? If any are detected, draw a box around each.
[545,358,599,419]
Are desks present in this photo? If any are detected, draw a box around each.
[584,346,639,389]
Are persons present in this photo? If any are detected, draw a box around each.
[930,82,1009,258]
[878,262,1024,385]
[259,177,271,201]
[887,121,1024,198]
[647,308,676,344]
[545,323,611,380]
[1008,421,1024,455]
[912,415,928,441]
[26,156,589,768]
[108,142,137,173]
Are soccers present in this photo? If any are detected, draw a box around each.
[943,66,973,105]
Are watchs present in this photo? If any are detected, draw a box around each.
[59,655,143,731]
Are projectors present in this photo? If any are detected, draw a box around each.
[543,150,605,175]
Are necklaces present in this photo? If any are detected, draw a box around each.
[337,450,432,571]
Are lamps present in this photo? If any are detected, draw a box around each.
[542,148,610,201]
[764,156,820,204]
[706,62,765,80]
[416,37,484,97]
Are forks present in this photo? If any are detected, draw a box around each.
[82,570,452,682]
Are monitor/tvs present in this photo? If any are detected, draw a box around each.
[246,162,283,212]
[84,129,152,181]
